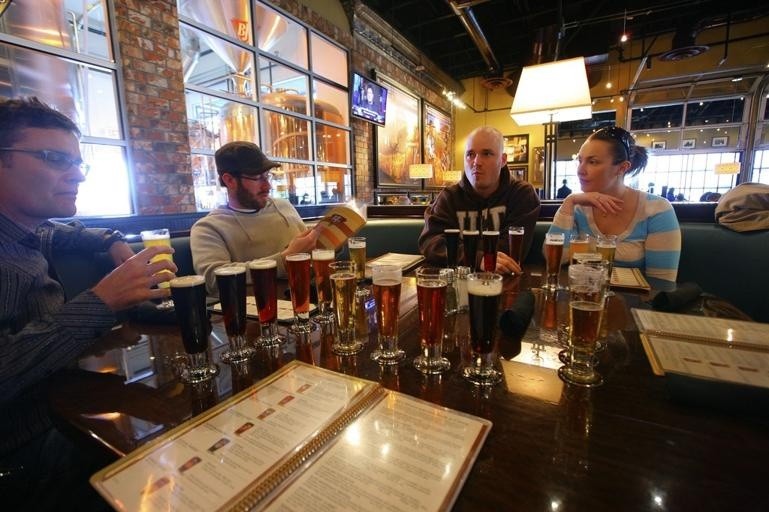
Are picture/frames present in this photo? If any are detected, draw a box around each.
[408,192,432,205]
[507,165,528,185]
[651,140,666,150]
[421,96,452,190]
[680,138,696,149]
[432,192,440,204]
[374,191,408,205]
[711,136,729,148]
[373,68,423,189]
[531,146,544,185]
[503,134,529,164]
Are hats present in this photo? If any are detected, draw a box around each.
[215,142,282,175]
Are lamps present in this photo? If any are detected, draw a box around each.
[408,163,433,189]
[443,171,462,185]
[509,56,593,200]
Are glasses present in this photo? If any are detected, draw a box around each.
[596,126,630,161]
[1,147,90,177]
[231,173,275,183]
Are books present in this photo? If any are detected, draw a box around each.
[607,264,653,290]
[363,252,426,281]
[630,306,769,390]
[300,197,368,258]
[90,358,491,510]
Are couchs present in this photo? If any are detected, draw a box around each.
[48,218,768,324]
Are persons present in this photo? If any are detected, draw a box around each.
[0,97,176,512]
[420,127,540,275]
[668,187,676,202]
[544,126,684,280]
[557,179,573,200]
[343,281,343,287]
[359,84,381,113]
[190,144,316,292]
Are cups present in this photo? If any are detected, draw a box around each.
[480,232,501,272]
[330,258,353,274]
[140,227,175,309]
[247,260,291,351]
[313,250,338,327]
[331,272,364,355]
[542,232,565,296]
[446,229,463,271]
[348,240,367,284]
[414,266,450,374]
[463,275,504,386]
[536,284,573,346]
[285,253,317,337]
[214,263,255,363]
[509,228,526,272]
[168,274,219,385]
[371,265,408,365]
[462,229,480,272]
[560,234,620,392]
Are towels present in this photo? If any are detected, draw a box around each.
[652,279,703,312]
[131,302,211,329]
[284,277,324,301]
[498,289,535,336]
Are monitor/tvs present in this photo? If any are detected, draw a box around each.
[348,69,388,128]
